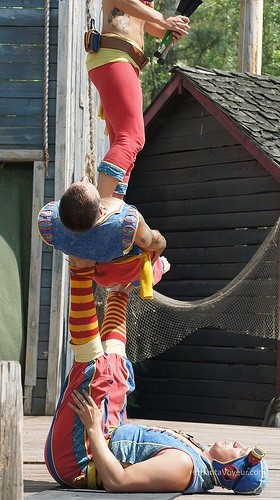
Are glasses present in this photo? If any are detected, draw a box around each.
[232,446,266,491]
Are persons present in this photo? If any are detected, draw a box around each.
[44,255,267,495]
[35,183,170,289]
[86,0,191,203]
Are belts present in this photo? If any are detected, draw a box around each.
[101,34,148,69]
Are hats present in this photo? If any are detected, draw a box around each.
[213,455,269,495]
